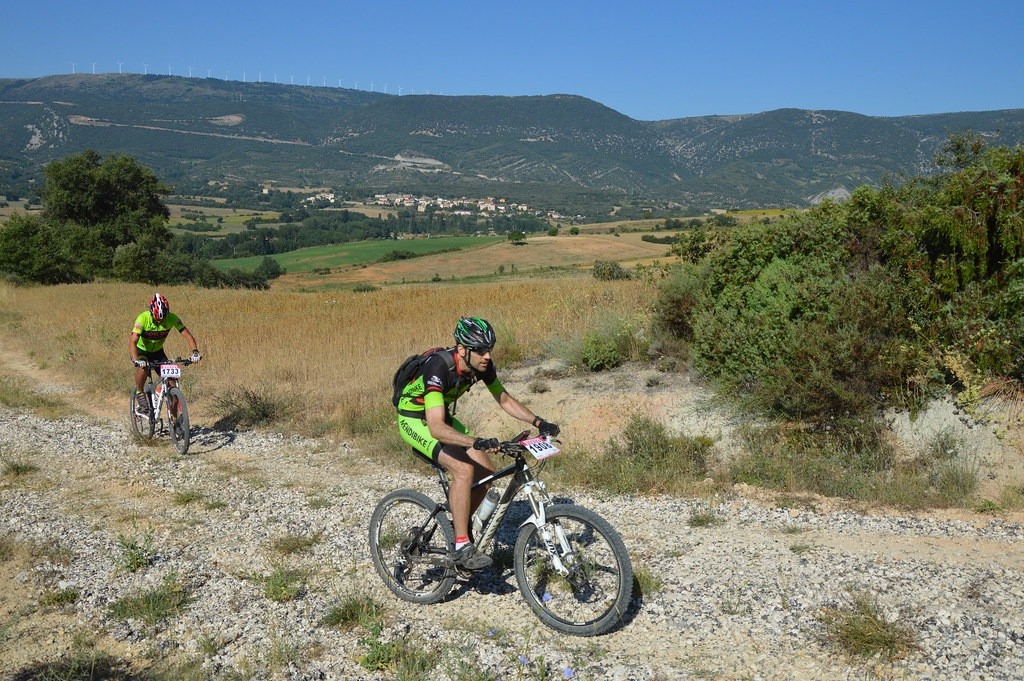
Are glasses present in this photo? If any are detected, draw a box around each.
[467,346,493,356]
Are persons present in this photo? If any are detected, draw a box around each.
[129,293,201,435]
[398,317,561,570]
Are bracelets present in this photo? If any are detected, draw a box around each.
[533,416,542,427]
[193,349,198,352]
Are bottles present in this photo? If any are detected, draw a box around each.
[154,387,161,402]
[471,487,500,533]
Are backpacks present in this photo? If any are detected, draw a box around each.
[393,348,456,406]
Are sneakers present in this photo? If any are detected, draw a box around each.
[175,427,185,438]
[452,543,493,569]
[135,390,148,407]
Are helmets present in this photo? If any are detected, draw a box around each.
[453,317,497,350]
[149,293,169,323]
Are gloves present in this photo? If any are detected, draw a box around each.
[134,360,152,369]
[188,353,202,364]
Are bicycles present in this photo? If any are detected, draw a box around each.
[130,355,203,455]
[368,432,633,637]
